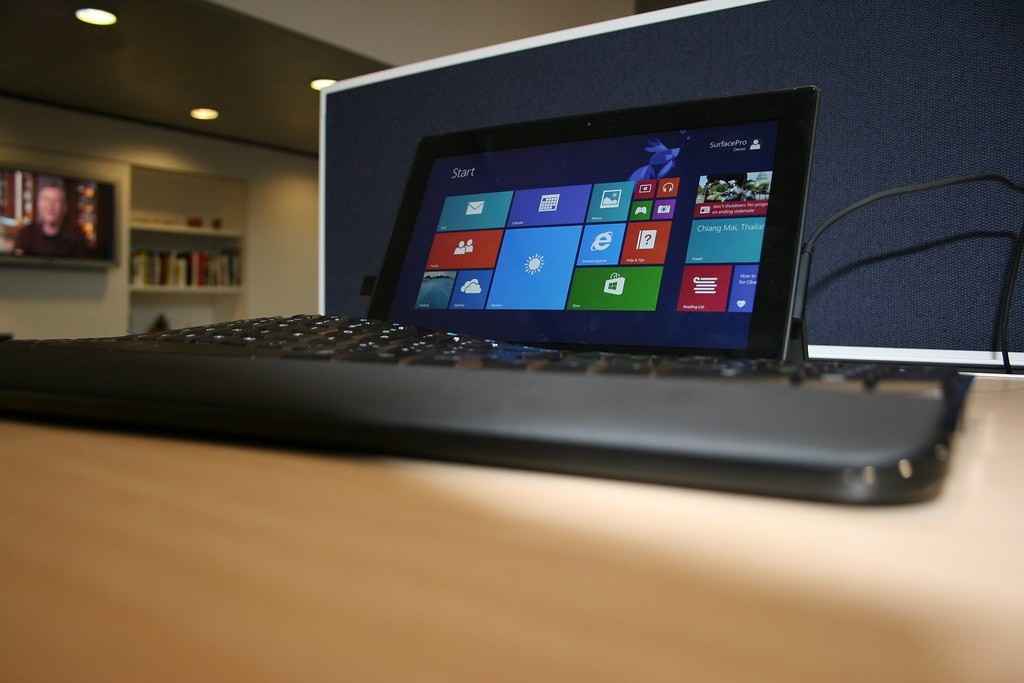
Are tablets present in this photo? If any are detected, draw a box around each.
[372,85,821,362]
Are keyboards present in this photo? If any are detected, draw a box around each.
[0,313,967,504]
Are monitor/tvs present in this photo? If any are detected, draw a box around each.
[0,164,120,268]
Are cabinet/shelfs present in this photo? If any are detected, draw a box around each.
[127,214,245,294]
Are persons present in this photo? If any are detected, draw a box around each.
[705,188,723,201]
[11,184,92,260]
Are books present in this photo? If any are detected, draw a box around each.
[130,249,238,287]
